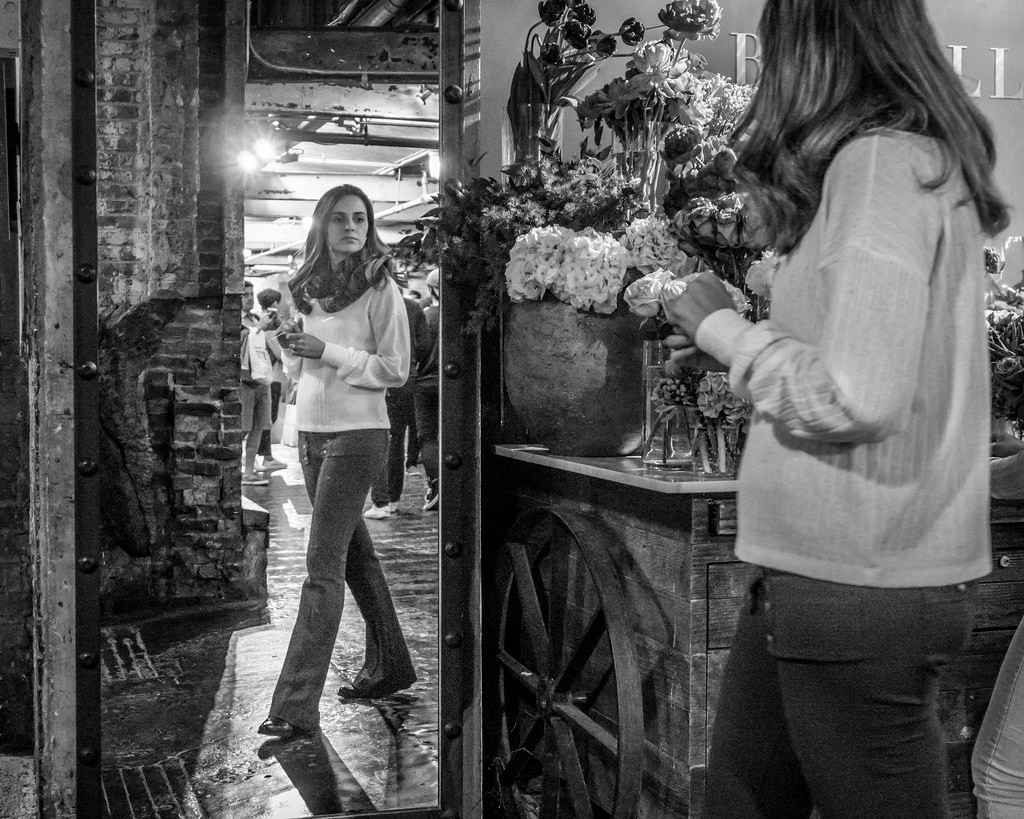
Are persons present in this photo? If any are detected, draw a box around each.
[657,0,1019,819]
[243,266,439,521]
[255,183,420,740]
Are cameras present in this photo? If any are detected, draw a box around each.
[269,313,273,319]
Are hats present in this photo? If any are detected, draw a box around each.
[427,269,440,296]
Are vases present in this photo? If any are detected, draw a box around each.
[694,416,742,476]
[640,332,700,469]
[502,299,660,457]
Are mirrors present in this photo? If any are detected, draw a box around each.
[69,0,465,819]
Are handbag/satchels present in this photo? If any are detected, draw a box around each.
[248,327,273,385]
[281,390,300,446]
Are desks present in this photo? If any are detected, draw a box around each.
[483,444,1024,819]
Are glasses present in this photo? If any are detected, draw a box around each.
[275,299,280,304]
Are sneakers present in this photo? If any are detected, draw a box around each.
[241,455,268,471]
[241,472,270,485]
[423,478,439,510]
[406,466,421,476]
[262,457,287,469]
[364,502,399,519]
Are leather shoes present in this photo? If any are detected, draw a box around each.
[338,681,411,699]
[257,716,299,737]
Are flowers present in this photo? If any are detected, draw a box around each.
[388,0,1024,473]
